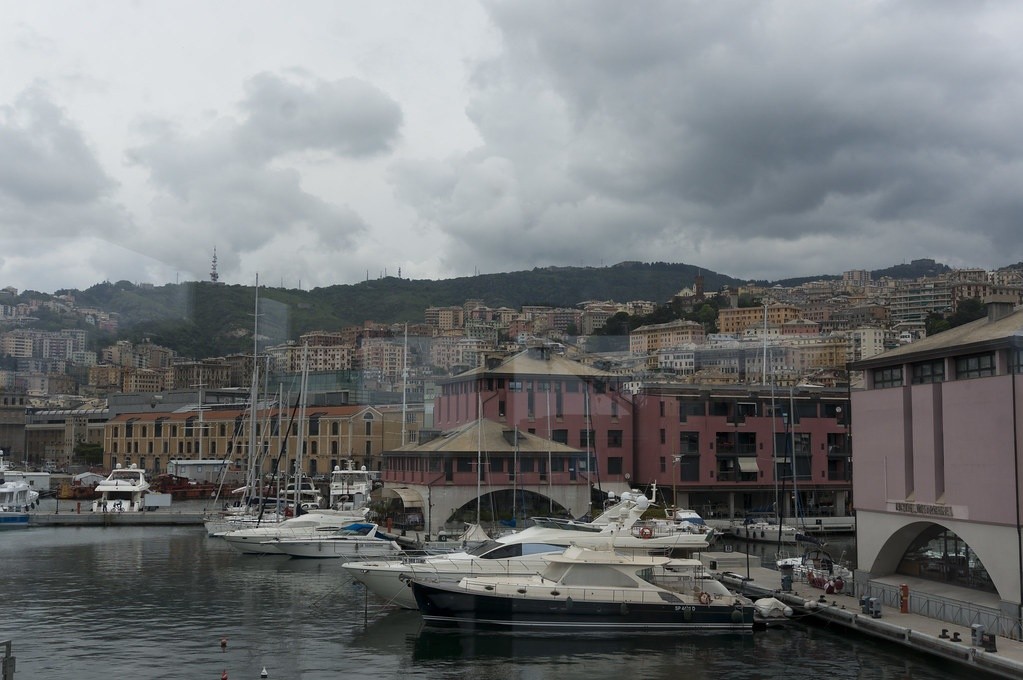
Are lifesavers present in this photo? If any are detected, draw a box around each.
[699,591,711,604]
[640,528,653,539]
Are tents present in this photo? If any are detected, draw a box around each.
[458,524,493,542]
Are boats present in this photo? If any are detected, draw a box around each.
[89,462,152,513]
[0,480,39,530]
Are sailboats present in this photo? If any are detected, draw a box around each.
[729,344,807,544]
[203,271,794,636]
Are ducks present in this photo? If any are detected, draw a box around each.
[218,638,229,649]
[260,666,268,678]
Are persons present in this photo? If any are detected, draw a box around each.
[274,506,277,512]
[366,511,372,520]
[116,500,123,509]
[225,503,229,510]
[102,498,108,512]
[282,506,292,516]
